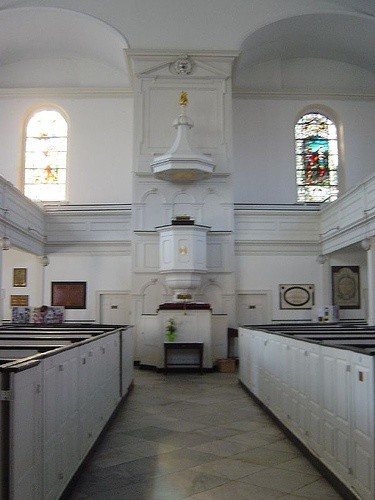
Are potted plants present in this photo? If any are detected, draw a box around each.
[165,320,178,342]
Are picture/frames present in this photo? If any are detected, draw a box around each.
[10,294,29,306]
[279,284,315,310]
[51,281,86,310]
[330,265,360,309]
[13,268,27,287]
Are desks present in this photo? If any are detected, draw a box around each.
[162,341,205,375]
